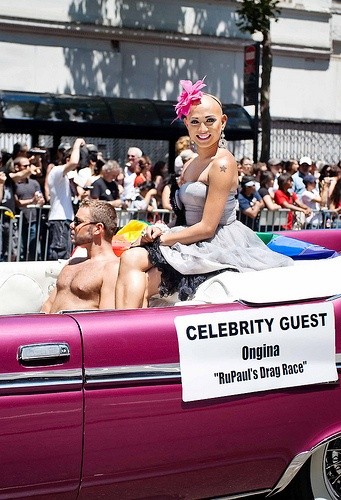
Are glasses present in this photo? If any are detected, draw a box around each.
[74,217,96,227]
[18,164,31,168]
[127,155,138,157]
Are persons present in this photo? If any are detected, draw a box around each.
[114,80,295,310]
[39,198,121,313]
[0,136,341,262]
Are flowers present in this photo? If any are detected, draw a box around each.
[169,75,207,124]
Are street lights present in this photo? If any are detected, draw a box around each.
[244,30,263,162]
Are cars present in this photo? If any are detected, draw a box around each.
[0,228,341,500]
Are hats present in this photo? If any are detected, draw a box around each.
[303,175,317,185]
[242,176,255,186]
[180,149,198,161]
[268,158,281,167]
[299,156,312,165]
